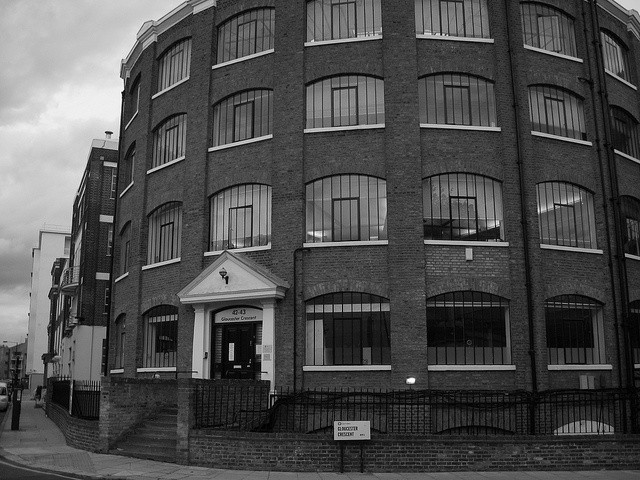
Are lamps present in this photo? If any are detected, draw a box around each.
[219,267,229,284]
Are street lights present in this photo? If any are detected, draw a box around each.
[3,341,18,400]
